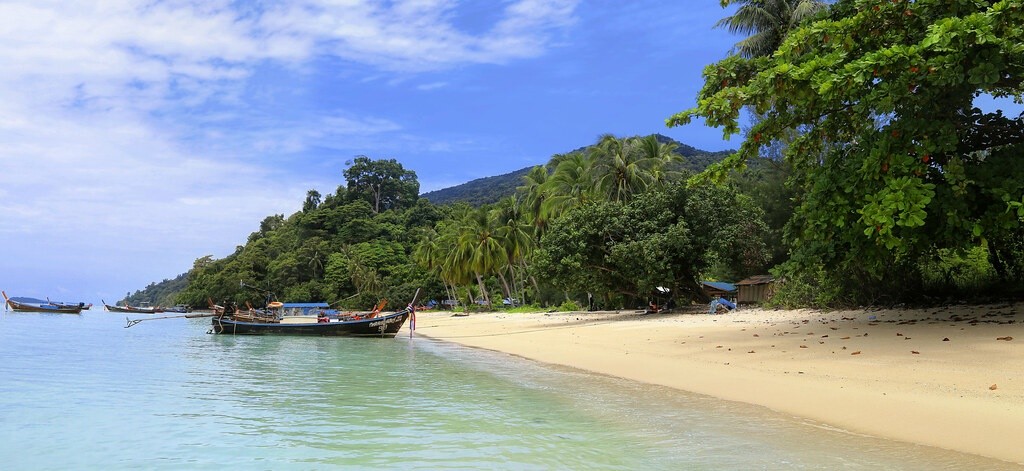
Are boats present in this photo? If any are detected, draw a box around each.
[102,299,165,314]
[2,291,89,314]
[212,287,423,338]
